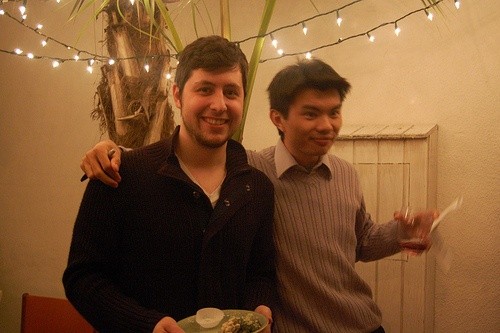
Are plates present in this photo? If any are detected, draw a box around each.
[176,310,269,333]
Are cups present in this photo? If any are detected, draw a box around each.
[393,204,434,256]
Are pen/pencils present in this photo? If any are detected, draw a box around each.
[80,148,116,183]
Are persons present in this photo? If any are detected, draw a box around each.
[62,35,275,333]
[79,54,441,333]
